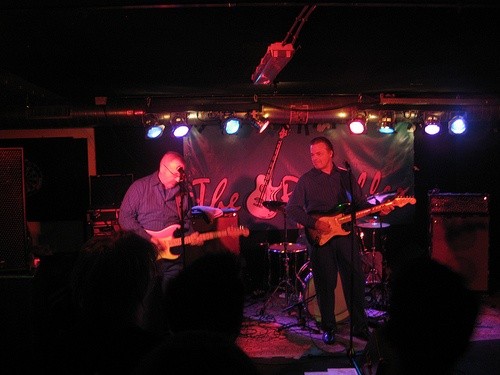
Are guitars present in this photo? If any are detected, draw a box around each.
[304,196,416,249]
[143,224,250,259]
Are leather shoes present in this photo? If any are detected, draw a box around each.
[322,329,336,345]
[353,330,370,341]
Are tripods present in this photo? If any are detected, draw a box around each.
[259,171,364,375]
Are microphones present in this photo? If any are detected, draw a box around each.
[343,160,352,172]
[176,165,185,173]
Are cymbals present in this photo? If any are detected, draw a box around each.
[356,222,391,228]
[262,200,287,215]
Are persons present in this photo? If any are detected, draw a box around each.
[120,152,203,293]
[286,136,395,344]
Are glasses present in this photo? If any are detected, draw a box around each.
[165,165,181,179]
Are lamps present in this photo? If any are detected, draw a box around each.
[142,95,270,140]
[347,94,468,135]
[251,4,320,85]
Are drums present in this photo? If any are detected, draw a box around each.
[268,242,307,291]
[295,260,351,325]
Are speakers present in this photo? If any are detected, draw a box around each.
[0,147,29,279]
[361,326,387,375]
[426,214,492,293]
